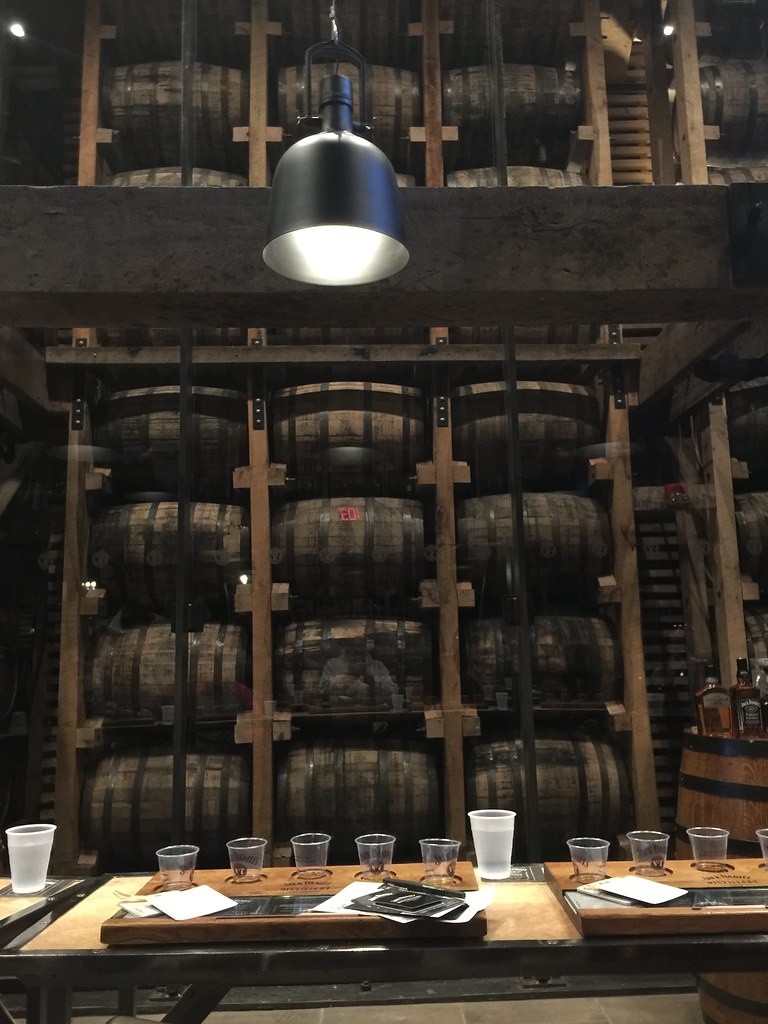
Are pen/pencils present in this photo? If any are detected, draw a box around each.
[382,877,466,899]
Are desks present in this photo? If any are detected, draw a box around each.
[0,859,763,1023]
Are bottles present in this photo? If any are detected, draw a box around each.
[735,671,763,735]
[694,664,732,737]
[757,659,768,731]
[729,658,760,735]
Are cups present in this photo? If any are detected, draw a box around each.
[419,839,461,888]
[468,809,517,880]
[355,834,396,880]
[156,844,200,892]
[755,829,768,870]
[686,827,730,871]
[626,831,670,877]
[291,833,331,879]
[226,837,268,882]
[5,824,57,894]
[567,837,610,884]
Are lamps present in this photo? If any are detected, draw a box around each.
[262,0,410,287]
